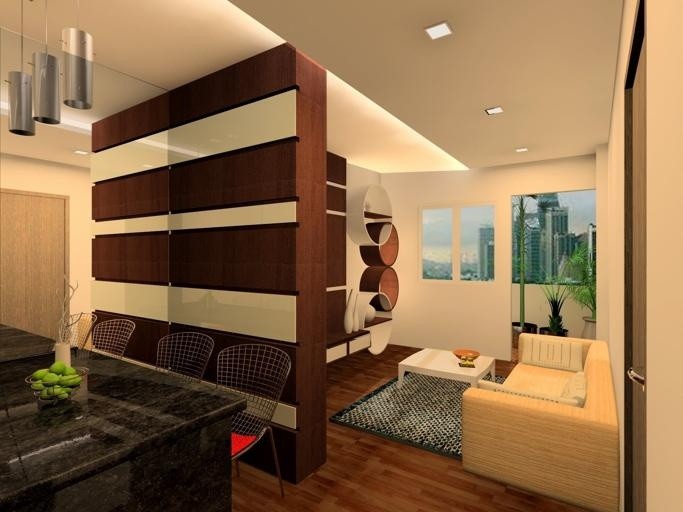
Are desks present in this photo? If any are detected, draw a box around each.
[0,323,246,512]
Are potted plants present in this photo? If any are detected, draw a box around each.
[566,242,597,339]
[521,261,570,336]
[512,194,537,348]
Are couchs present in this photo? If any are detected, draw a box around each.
[462,333,620,511]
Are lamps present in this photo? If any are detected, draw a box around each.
[31,1,60,125]
[58,1,94,112]
[424,19,453,41]
[5,1,36,136]
[484,105,504,115]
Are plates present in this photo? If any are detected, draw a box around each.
[453,349,479,360]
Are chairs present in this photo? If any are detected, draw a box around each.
[155,331,215,382]
[216,344,287,498]
[61,313,96,350]
[90,318,136,357]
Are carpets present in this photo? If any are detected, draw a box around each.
[331,372,508,462]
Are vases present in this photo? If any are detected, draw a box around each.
[539,327,568,338]
[511,322,535,348]
[582,316,597,339]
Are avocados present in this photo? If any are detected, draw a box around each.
[32,361,81,400]
